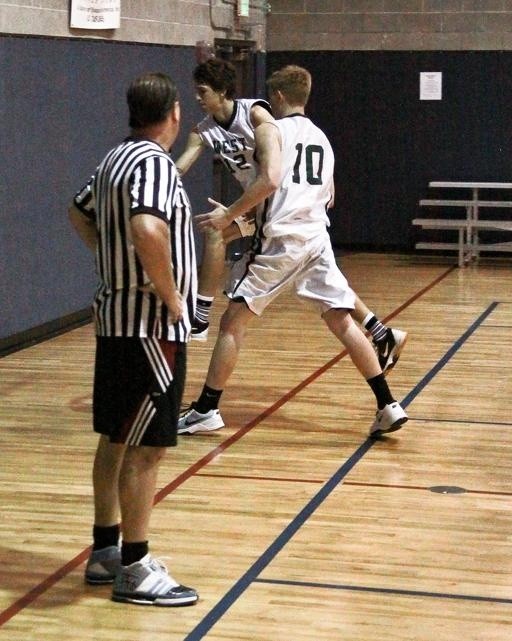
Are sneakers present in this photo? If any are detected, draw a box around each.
[111,553,198,605]
[372,328,408,376]
[191,316,209,341]
[85,547,121,583]
[370,400,408,435]
[177,402,225,435]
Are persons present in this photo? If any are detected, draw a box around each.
[173,54,409,379]
[69,72,201,608]
[177,64,410,437]
